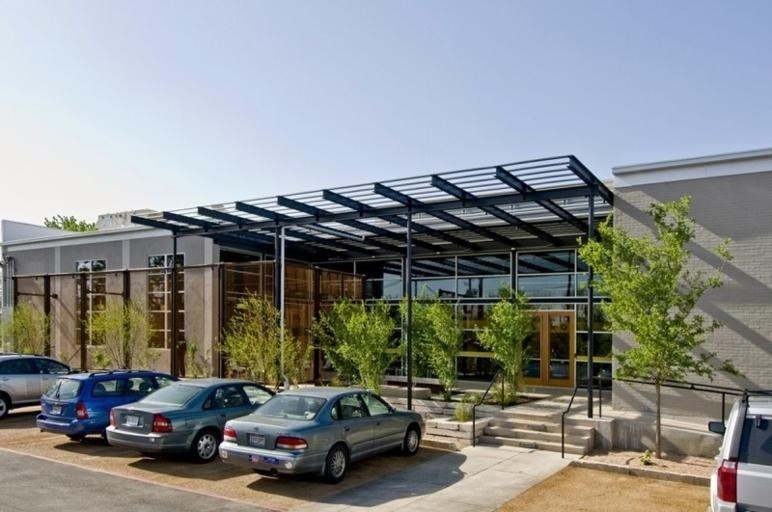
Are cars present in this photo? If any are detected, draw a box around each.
[106,377,276,464]
[218,386,425,484]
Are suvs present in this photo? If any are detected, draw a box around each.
[708,389,772,512]
[0,352,81,419]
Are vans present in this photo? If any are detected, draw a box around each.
[37,368,183,446]
